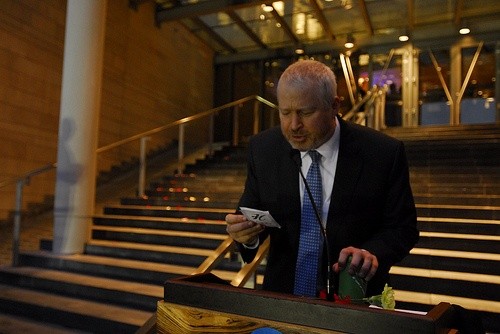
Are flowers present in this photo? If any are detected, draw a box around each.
[310,282,396,310]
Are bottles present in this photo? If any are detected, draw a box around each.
[338,254,368,304]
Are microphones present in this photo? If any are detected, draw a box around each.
[289,149,332,301]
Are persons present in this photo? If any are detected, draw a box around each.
[224,58,421,307]
[354,77,402,126]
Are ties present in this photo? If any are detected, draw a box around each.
[294,150,323,297]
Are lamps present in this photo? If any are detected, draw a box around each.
[398,27,408,41]
[458,18,470,35]
[344,34,354,49]
